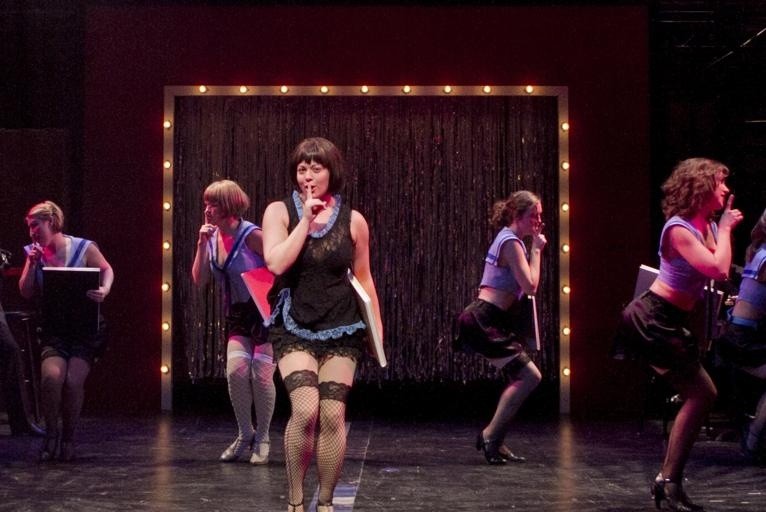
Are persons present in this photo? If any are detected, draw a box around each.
[719,210,765,465]
[615,157,743,512]
[191,179,276,465]
[0,247,45,436]
[18,200,114,463]
[262,136,384,511]
[456,190,548,465]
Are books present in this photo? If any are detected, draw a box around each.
[345,268,389,369]
[634,263,725,340]
[240,266,275,321]
[41,263,100,330]
[511,295,542,352]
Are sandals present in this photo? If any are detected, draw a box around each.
[316,500,334,512]
[289,497,304,512]
[740,428,766,464]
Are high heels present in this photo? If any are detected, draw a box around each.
[218,430,257,463]
[249,433,271,465]
[476,430,507,465]
[651,470,703,510]
[499,444,527,463]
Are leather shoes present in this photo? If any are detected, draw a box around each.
[42,430,60,460]
[10,421,46,437]
[61,436,76,464]
